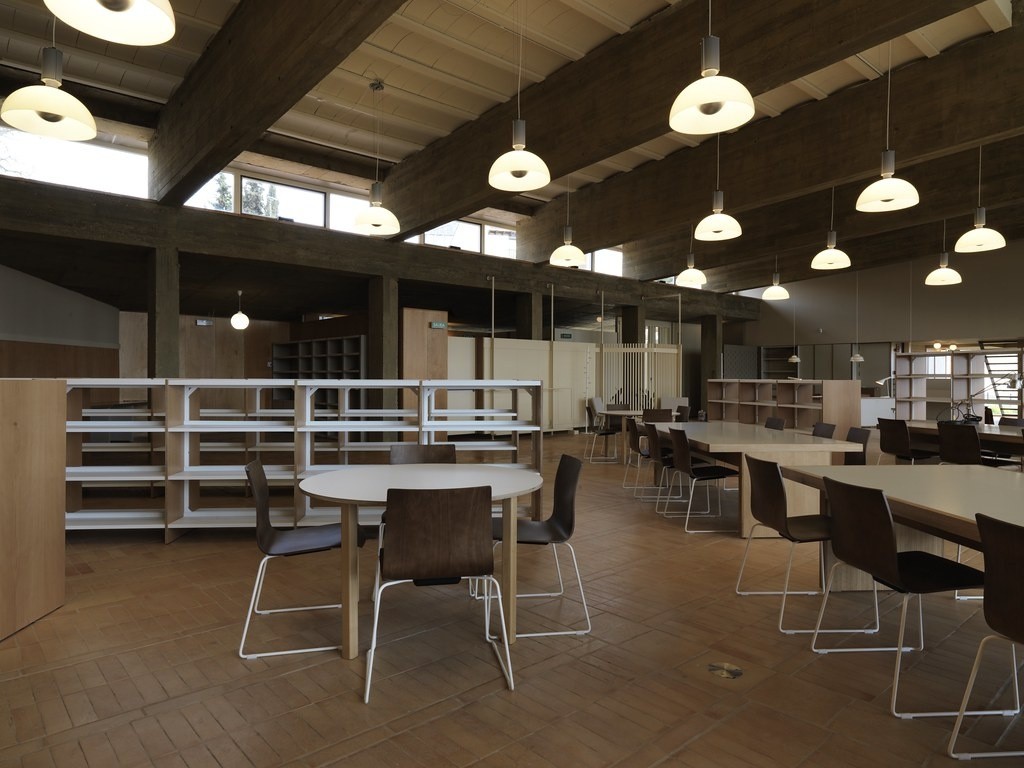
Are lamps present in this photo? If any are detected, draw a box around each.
[810,184,852,270]
[353,80,400,235]
[855,42,920,213]
[788,304,802,363]
[694,132,743,241]
[43,0,175,46]
[761,254,790,300]
[850,271,865,362]
[549,175,587,267]
[668,0,756,135]
[0,15,97,141]
[925,218,962,286]
[675,225,707,288]
[229,290,250,331]
[487,0,551,192]
[954,145,1007,253]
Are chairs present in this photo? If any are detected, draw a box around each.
[581,396,1024,768]
[236,460,363,661]
[363,486,516,703]
[489,452,592,640]
[390,444,457,464]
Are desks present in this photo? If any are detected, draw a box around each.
[780,464,1024,554]
[598,410,680,465]
[297,461,544,660]
[637,422,863,539]
[876,420,1024,449]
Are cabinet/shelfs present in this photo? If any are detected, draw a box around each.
[706,378,861,466]
[271,334,368,441]
[62,378,545,548]
[895,349,1024,424]
